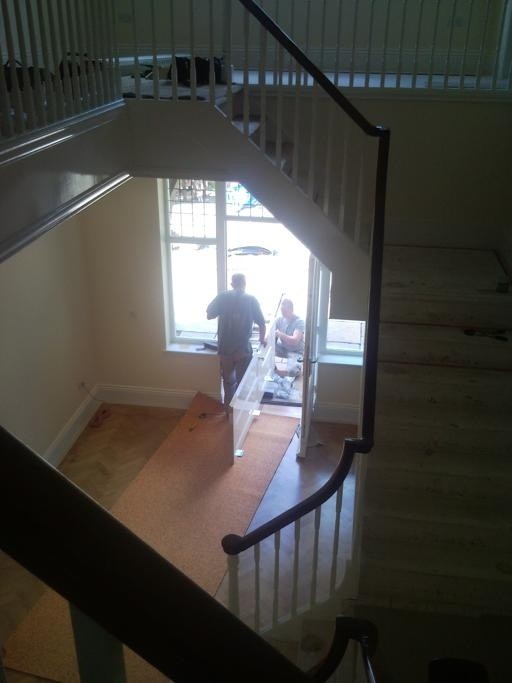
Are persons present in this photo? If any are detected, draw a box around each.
[275,299,306,377]
[206,273,267,408]
